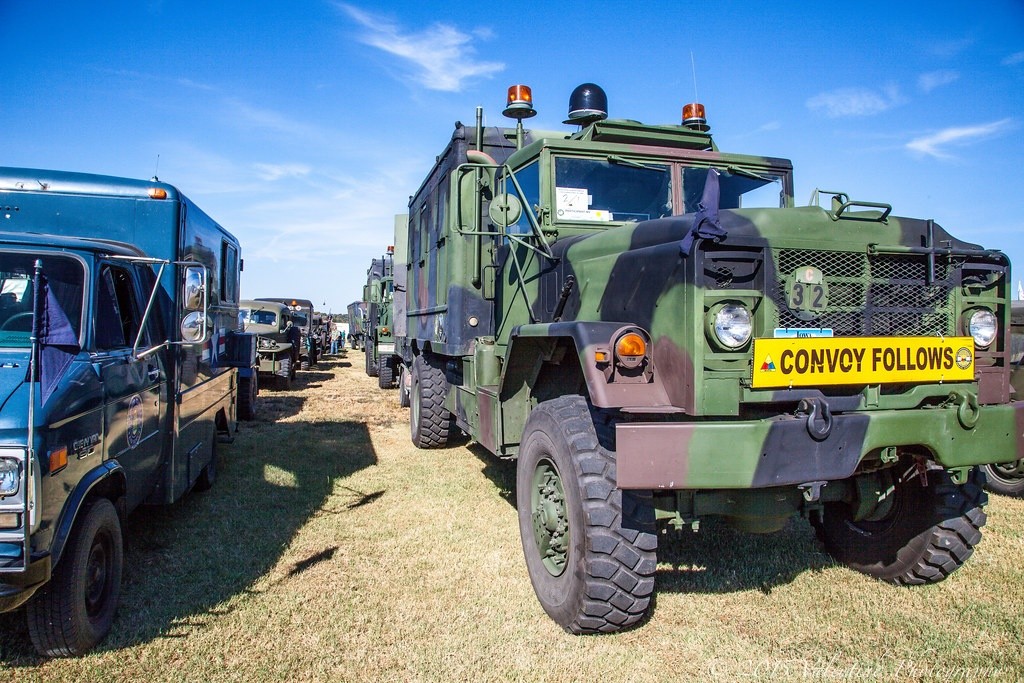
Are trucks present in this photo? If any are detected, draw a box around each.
[0,168,259,659]
[232,208,405,392]
[393,81,1021,634]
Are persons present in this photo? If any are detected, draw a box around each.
[327,324,340,355]
